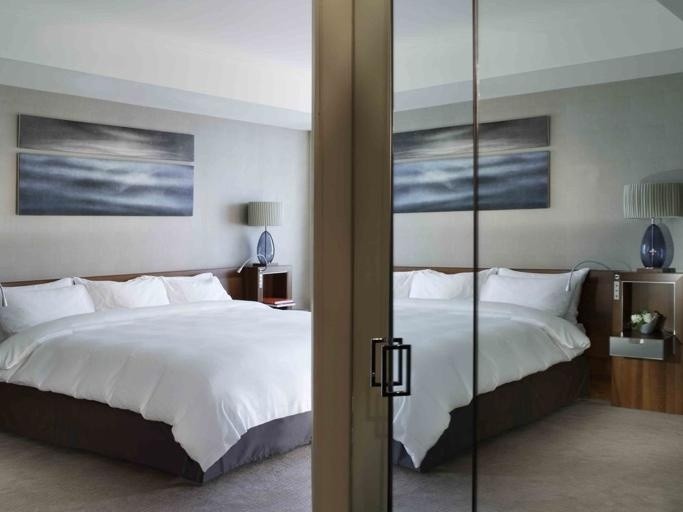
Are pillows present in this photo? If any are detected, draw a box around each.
[392,271,413,298]
[1,273,235,335]
[484,266,591,322]
[411,266,496,300]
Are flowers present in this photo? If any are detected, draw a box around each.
[629,309,654,327]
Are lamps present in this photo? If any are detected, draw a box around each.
[565,260,620,299]
[237,254,268,289]
[247,201,285,266]
[622,182,683,273]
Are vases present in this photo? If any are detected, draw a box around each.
[640,315,661,333]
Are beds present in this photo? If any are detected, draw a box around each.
[1,265,313,484]
[389,264,597,474]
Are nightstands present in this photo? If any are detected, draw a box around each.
[609,270,683,415]
[244,263,292,310]
